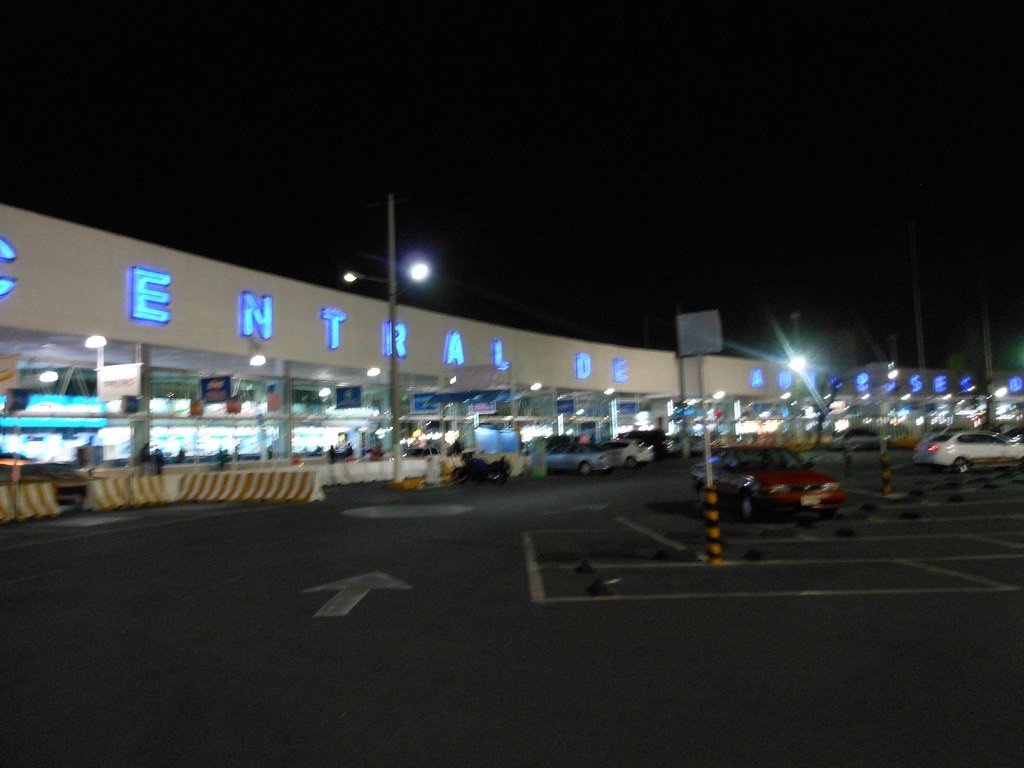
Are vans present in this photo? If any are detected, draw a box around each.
[616,430,668,459]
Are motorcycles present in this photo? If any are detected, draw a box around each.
[450,450,511,486]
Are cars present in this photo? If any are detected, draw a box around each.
[690,446,846,520]
[382,446,441,461]
[832,429,889,454]
[666,436,704,452]
[530,442,613,476]
[911,425,1023,473]
[601,440,654,468]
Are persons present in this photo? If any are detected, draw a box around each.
[154,449,165,474]
[141,442,150,462]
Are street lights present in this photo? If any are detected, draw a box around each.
[342,272,400,485]
